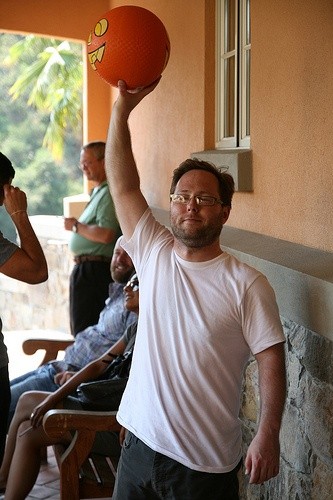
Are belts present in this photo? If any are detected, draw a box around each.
[73,255,112,265]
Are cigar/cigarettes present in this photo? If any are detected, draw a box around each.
[19,425,33,437]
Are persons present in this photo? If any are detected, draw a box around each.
[4,234,137,433]
[103,75,288,499]
[0,272,139,500]
[63,141,126,337]
[0,149,48,495]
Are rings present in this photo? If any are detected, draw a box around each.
[31,413,35,418]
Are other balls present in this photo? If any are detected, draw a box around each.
[89,4,171,92]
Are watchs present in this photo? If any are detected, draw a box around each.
[72,222,81,232]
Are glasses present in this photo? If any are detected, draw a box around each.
[77,160,96,169]
[169,193,225,207]
[126,281,140,292]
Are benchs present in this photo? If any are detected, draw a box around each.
[21,336,123,500]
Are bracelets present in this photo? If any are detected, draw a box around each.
[11,210,26,216]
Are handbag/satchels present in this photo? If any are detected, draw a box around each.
[77,377,126,410]
